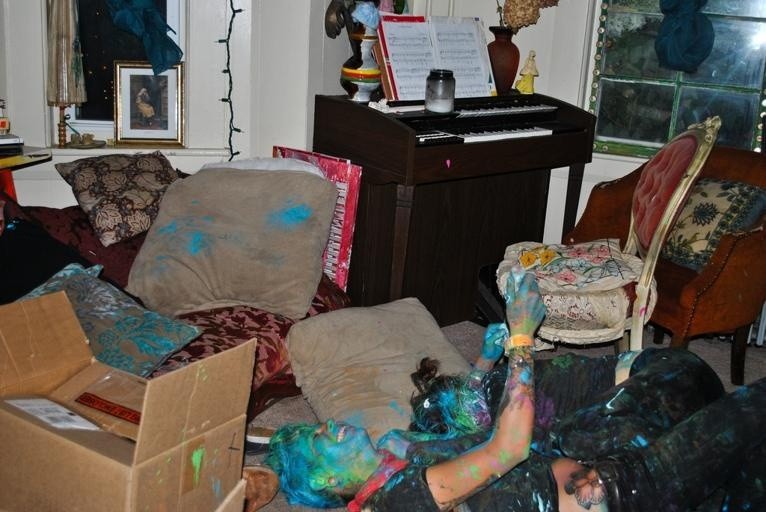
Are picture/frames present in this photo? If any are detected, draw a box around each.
[113,61,187,150]
[40,0,187,148]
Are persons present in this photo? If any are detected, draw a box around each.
[136,86,155,126]
[261,271,766,512]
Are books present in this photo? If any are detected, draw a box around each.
[370,14,498,101]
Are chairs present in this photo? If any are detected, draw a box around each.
[559,141,766,386]
[495,114,721,354]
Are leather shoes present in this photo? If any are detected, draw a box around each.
[242,464,280,512]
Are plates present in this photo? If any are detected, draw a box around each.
[68,141,106,148]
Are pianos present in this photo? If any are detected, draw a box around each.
[313,92,596,329]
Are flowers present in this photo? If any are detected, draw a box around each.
[496,0,559,34]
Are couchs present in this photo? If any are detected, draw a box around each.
[0,191,351,395]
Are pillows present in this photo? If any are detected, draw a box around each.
[659,178,766,277]
[125,167,337,325]
[202,158,327,179]
[54,152,177,249]
[14,262,201,378]
[285,297,476,447]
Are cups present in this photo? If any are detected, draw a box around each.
[70,134,93,145]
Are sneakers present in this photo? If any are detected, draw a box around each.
[245,428,277,455]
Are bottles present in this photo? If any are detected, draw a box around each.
[425,68,455,114]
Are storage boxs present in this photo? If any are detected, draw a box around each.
[0,290,256,512]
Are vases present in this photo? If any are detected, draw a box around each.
[340,34,382,103]
[487,26,519,95]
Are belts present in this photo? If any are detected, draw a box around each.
[594,460,623,512]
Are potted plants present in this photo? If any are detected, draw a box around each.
[0,99,9,130]
